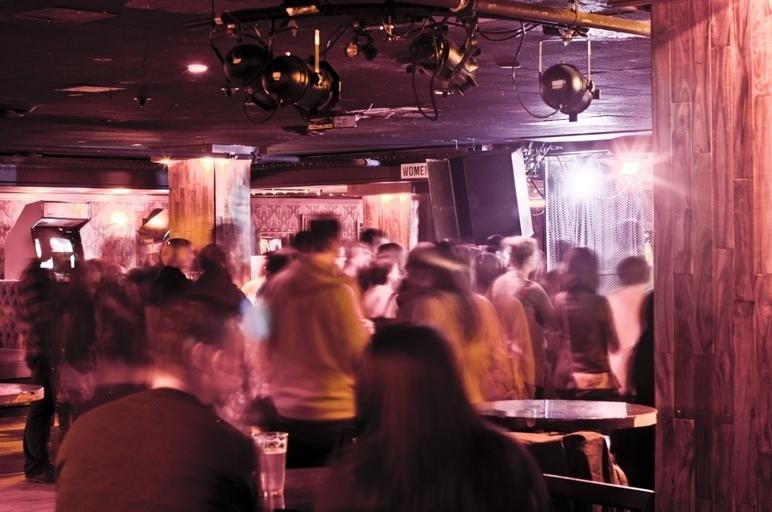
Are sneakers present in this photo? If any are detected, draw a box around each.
[25,464,56,484]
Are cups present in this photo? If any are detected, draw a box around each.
[252,432,289,498]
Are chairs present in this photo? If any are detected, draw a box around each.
[542,431,654,511]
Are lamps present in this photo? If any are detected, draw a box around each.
[181,0,651,124]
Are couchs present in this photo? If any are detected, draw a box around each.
[0,281,34,378]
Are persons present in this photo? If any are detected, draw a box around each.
[315,320,550,510]
[11,212,654,512]
[55,298,267,511]
